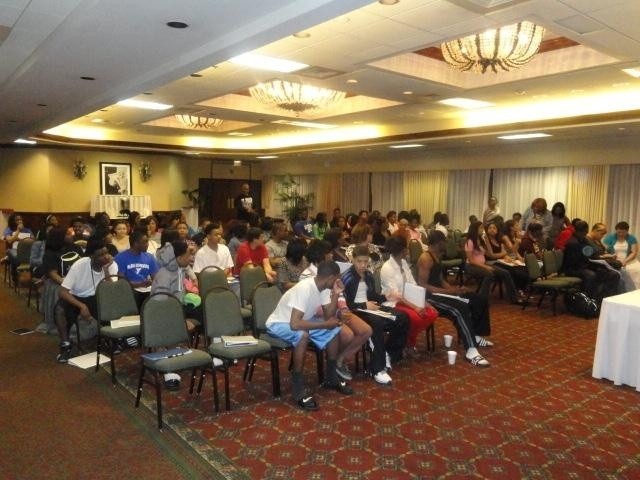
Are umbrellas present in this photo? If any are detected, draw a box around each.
[1,257,7,265]
[510,297,531,305]
[511,289,527,300]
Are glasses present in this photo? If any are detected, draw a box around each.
[441,20,546,75]
[175,113,224,131]
[248,80,346,114]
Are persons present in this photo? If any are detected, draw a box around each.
[339,245,410,384]
[264,260,353,410]
[464,197,638,318]
[236,184,256,220]
[294,207,450,260]
[346,222,387,295]
[414,230,495,367]
[379,234,439,359]
[36,212,351,347]
[54,239,121,363]
[5,214,36,283]
[298,239,372,380]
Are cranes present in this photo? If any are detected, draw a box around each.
[221,335,259,348]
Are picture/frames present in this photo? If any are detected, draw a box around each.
[88,195,153,221]
[593,288,640,392]
[99,161,133,196]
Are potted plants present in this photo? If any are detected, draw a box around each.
[99,161,133,196]
[273,175,315,229]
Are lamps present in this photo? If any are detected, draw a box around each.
[175,113,224,131]
[441,20,546,75]
[248,80,346,114]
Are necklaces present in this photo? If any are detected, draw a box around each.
[90,258,104,294]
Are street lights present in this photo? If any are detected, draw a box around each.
[61,251,82,277]
[564,290,601,318]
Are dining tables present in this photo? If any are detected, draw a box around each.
[273,175,315,229]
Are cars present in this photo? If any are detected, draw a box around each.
[447,351,458,364]
[444,334,453,347]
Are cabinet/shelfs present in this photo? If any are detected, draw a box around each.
[338,292,346,310]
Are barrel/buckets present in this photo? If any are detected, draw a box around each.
[463,354,490,367]
[476,337,493,349]
[35,320,50,335]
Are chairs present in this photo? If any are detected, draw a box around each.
[137,292,220,429]
[248,282,325,392]
[370,370,392,384]
[9,214,346,356]
[310,213,615,316]
[291,391,317,408]
[159,370,180,391]
[56,340,73,363]
[95,275,144,386]
[354,320,436,373]
[386,351,392,372]
[214,357,226,372]
[326,378,353,395]
[112,338,126,355]
[335,364,352,380]
[198,287,281,412]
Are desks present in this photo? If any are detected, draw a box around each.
[593,288,640,392]
[88,195,153,221]
